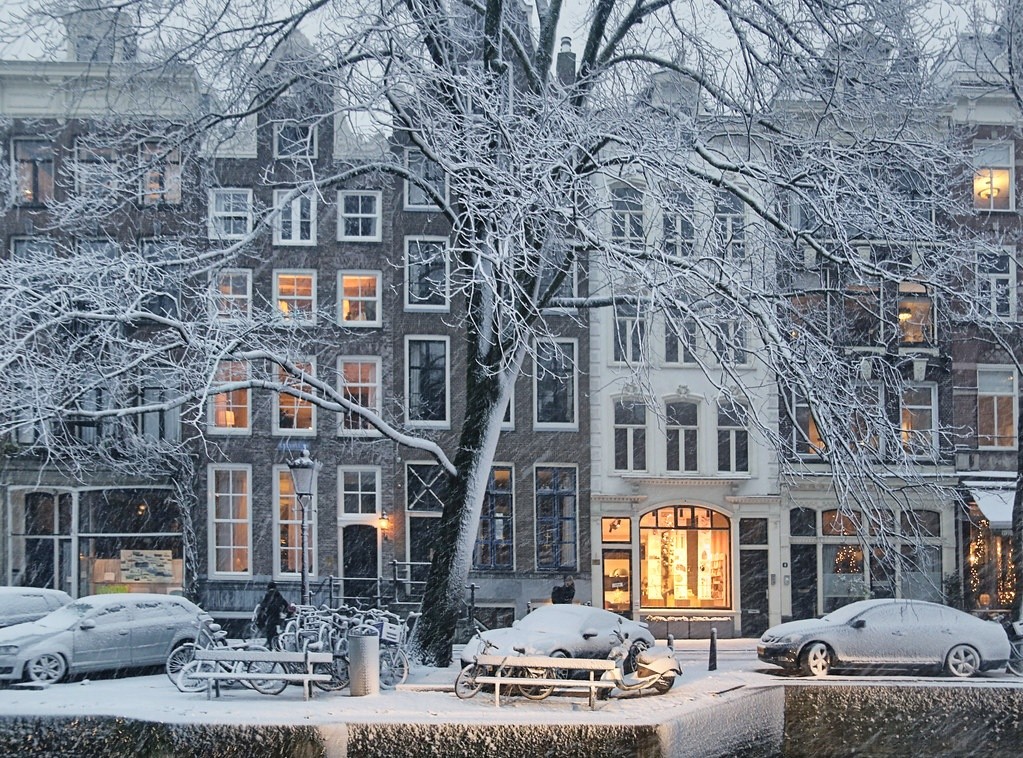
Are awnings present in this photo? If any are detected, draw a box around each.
[967,486,1015,530]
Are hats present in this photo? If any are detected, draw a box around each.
[268,582,276,589]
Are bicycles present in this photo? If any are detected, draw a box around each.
[166,598,424,696]
[982,609,1023,678]
[453,626,558,702]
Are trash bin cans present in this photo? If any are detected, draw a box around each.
[347,633,380,696]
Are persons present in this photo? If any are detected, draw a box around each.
[551,573,576,604]
[257,582,289,652]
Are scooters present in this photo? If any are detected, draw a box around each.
[596,618,682,699]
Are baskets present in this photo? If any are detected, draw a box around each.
[1013,621,1022,635]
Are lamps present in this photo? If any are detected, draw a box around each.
[940,353,953,373]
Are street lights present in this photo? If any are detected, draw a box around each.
[285,449,319,681]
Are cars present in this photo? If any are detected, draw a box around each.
[758,597,1012,677]
[0,585,219,687]
[463,602,655,677]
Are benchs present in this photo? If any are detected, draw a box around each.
[475,655,616,711]
[188,649,333,701]
